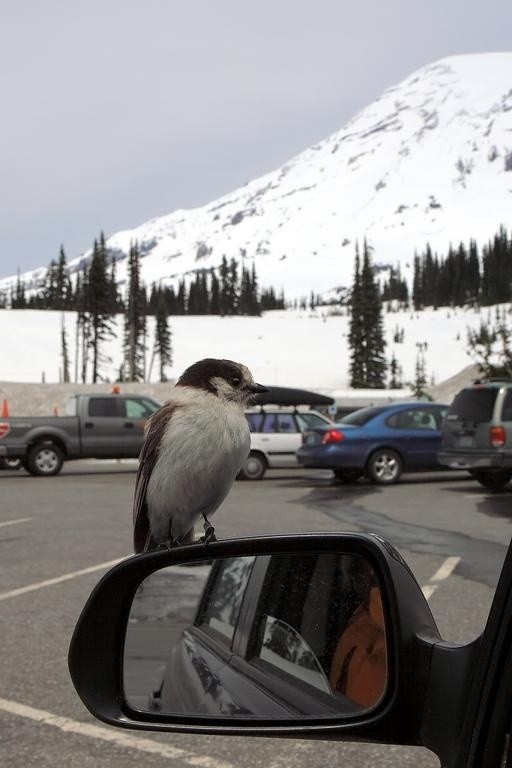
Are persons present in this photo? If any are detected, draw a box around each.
[330,570,387,708]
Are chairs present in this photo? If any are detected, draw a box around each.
[271,422,291,432]
[405,414,433,430]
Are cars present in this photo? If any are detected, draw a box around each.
[238,409,332,477]
[296,403,461,484]
[439,382,512,490]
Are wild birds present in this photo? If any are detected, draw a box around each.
[131,357,272,553]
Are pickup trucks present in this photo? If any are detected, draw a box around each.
[0,393,164,476]
